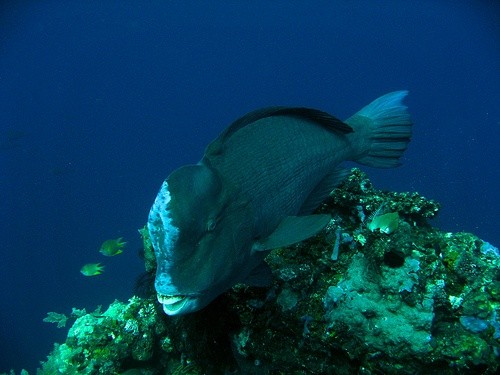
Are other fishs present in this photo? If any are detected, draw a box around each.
[146,90,411,315]
